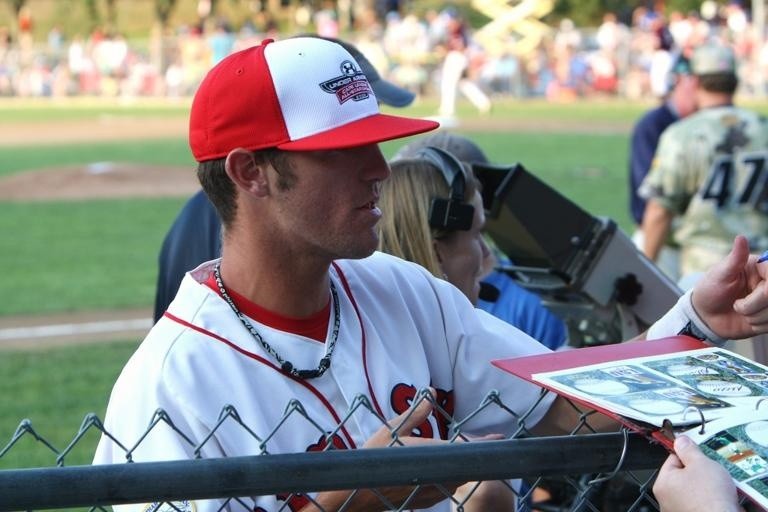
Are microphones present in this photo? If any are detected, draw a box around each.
[479,280,501,302]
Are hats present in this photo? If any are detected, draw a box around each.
[319,37,416,106]
[190,37,439,162]
[689,45,737,75]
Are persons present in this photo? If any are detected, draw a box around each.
[652,436,744,510]
[155,184,227,323]
[378,157,518,510]
[630,61,699,286]
[636,46,768,362]
[83,35,768,510]
[395,135,567,345]
[0,0,768,100]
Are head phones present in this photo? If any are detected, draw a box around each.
[415,145,475,232]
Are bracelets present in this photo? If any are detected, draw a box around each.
[644,293,722,344]
